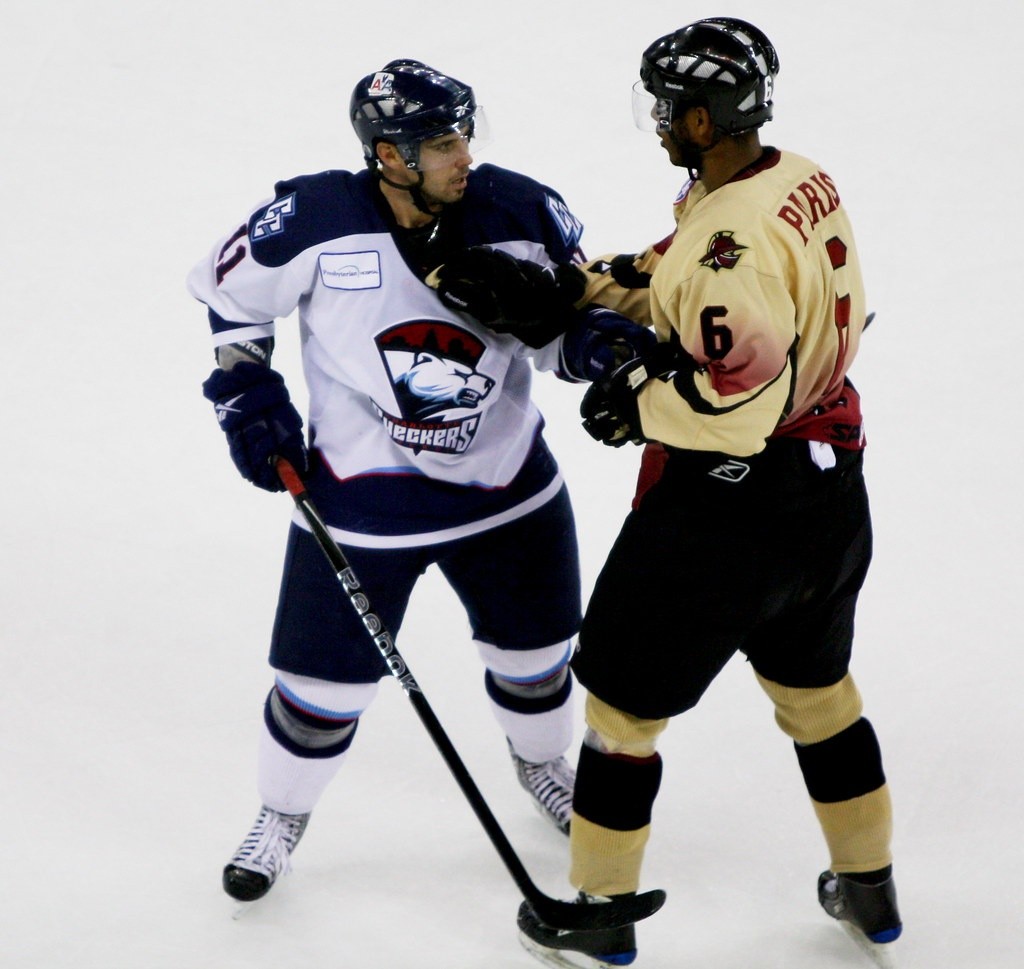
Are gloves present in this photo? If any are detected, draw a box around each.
[437,245,558,335]
[580,342,697,448]
[202,359,309,492]
[579,314,657,385]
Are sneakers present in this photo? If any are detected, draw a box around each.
[516,756,578,837]
[222,803,310,918]
[818,871,903,969]
[517,892,637,969]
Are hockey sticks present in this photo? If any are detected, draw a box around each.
[275,458,667,934]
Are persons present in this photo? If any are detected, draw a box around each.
[187,59,658,919]
[425,17,904,969]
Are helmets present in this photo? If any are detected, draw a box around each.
[349,58,477,160]
[632,16,780,136]
